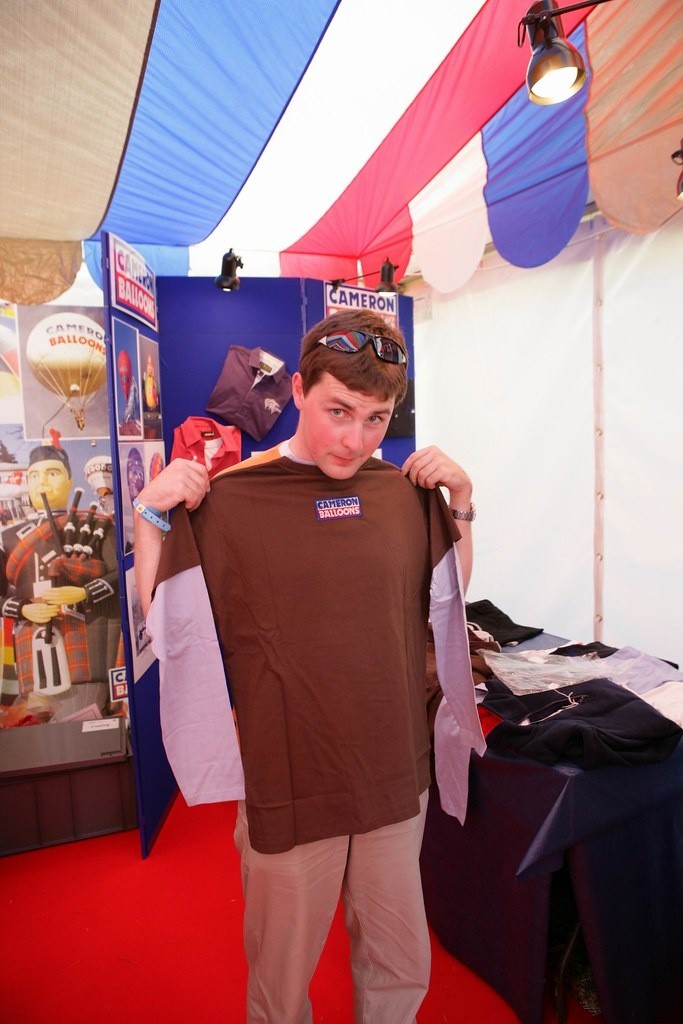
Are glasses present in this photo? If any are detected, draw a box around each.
[313,329,408,364]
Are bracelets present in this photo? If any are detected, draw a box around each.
[132,497,171,532]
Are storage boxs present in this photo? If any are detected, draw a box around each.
[0,714,141,859]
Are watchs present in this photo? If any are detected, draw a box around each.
[449,503,476,521]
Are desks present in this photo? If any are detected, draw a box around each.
[418,595,683,1024]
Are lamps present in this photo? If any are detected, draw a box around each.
[671,140,683,200]
[330,257,400,292]
[517,1,616,105]
[215,247,245,294]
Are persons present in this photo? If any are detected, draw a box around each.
[132,308,475,1024]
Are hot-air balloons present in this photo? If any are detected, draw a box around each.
[118,349,132,404]
[25,312,107,431]
[144,353,159,412]
